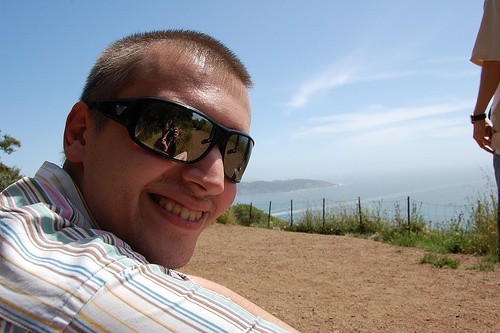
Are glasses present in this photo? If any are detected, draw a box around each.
[80,96,256,184]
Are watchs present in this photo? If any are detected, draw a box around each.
[470,114,486,124]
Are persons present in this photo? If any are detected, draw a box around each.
[0,29,301,333]
[154,119,187,160]
[470,0,491,264]
